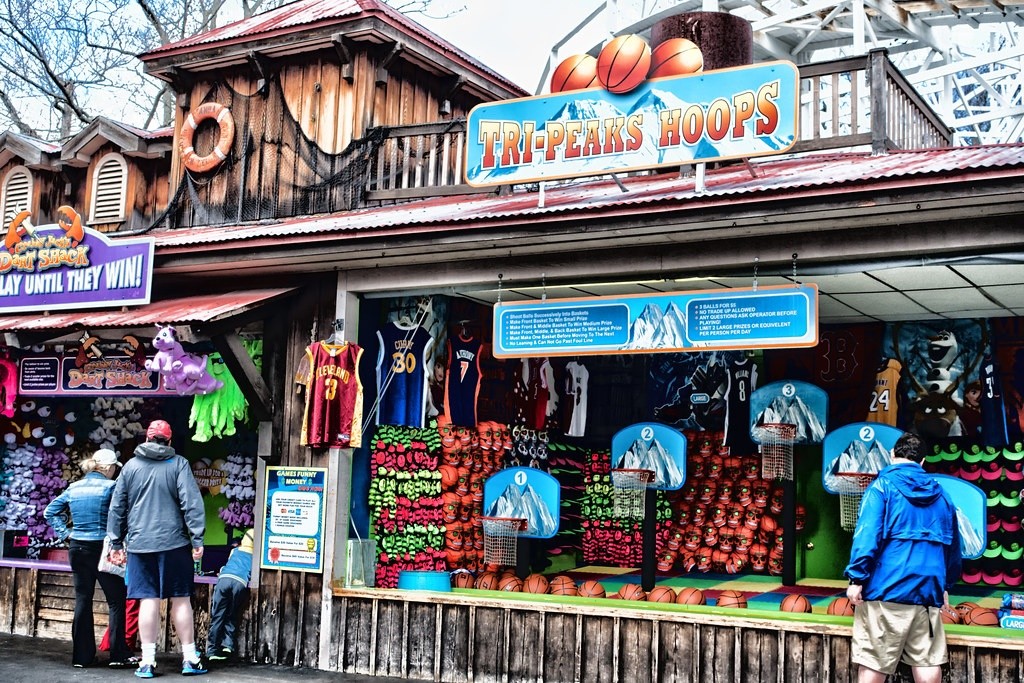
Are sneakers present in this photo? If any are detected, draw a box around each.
[134,665,153,678]
[182,658,208,676]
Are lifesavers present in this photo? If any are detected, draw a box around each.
[178,101,236,174]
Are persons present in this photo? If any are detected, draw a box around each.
[43,448,141,669]
[950,381,984,435]
[205,528,254,661]
[843,432,963,683]
[107,420,210,679]
[97,592,141,651]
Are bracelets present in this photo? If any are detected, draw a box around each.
[849,581,863,585]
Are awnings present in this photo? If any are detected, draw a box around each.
[0,282,295,412]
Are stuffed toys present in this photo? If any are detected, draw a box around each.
[0,325,263,574]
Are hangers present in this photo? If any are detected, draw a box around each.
[575,359,583,366]
[395,296,474,340]
[325,324,346,346]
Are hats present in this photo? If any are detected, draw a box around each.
[920,437,1024,586]
[92,449,123,467]
[146,419,172,442]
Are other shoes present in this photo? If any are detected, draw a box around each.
[73,656,98,668]
[209,655,231,665]
[108,656,142,670]
[220,647,231,657]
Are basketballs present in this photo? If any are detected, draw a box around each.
[827,597,855,616]
[939,601,999,628]
[644,37,705,80]
[550,53,601,92]
[454,570,749,611]
[595,33,653,96]
[778,593,812,613]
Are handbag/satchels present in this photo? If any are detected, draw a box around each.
[97,536,128,578]
